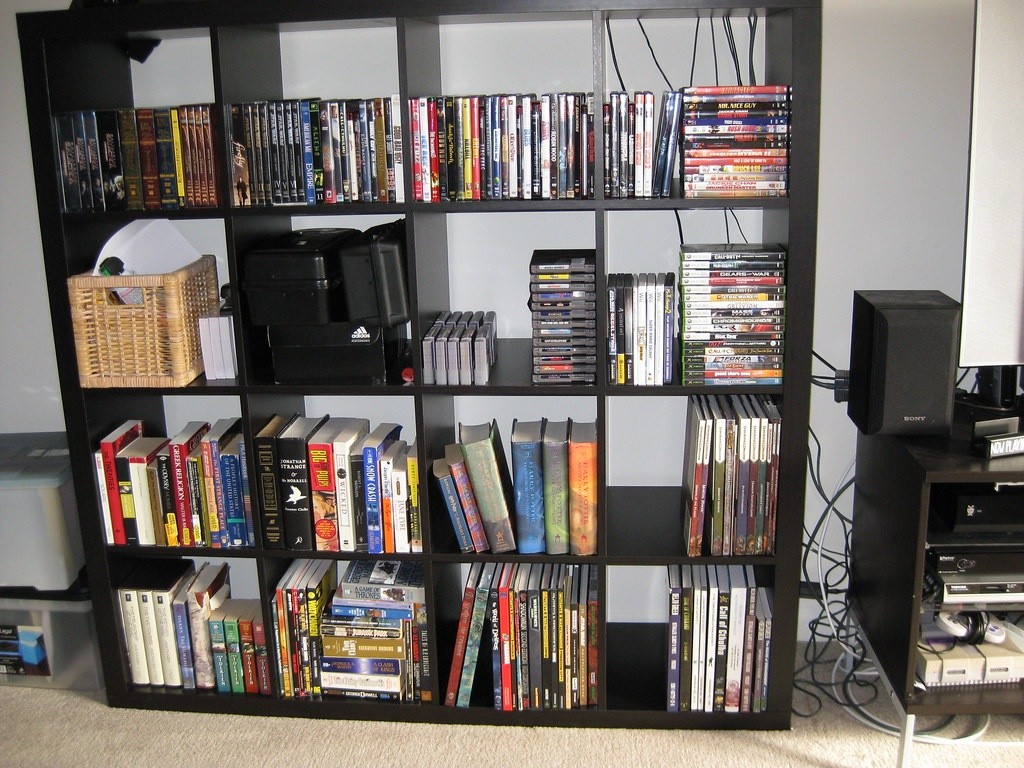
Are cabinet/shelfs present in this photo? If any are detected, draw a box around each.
[16,0,821,732]
[849,428,1024,768]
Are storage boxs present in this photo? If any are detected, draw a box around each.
[0,565,106,693]
[0,431,86,592]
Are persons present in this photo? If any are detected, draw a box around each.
[236,177,248,206]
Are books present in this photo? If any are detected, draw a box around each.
[53,103,218,214]
[117,559,271,695]
[529,243,786,390]
[666,565,772,713]
[423,310,497,385]
[228,92,405,206]
[602,85,787,197]
[444,562,598,710]
[270,559,432,701]
[682,394,781,557]
[94,417,255,548]
[431,417,597,555]
[252,413,423,554]
[407,92,595,202]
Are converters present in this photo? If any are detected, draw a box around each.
[799,581,828,601]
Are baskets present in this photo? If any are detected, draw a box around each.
[66,253,221,389]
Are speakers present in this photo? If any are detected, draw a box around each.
[976,365,1017,409]
[846,290,961,437]
